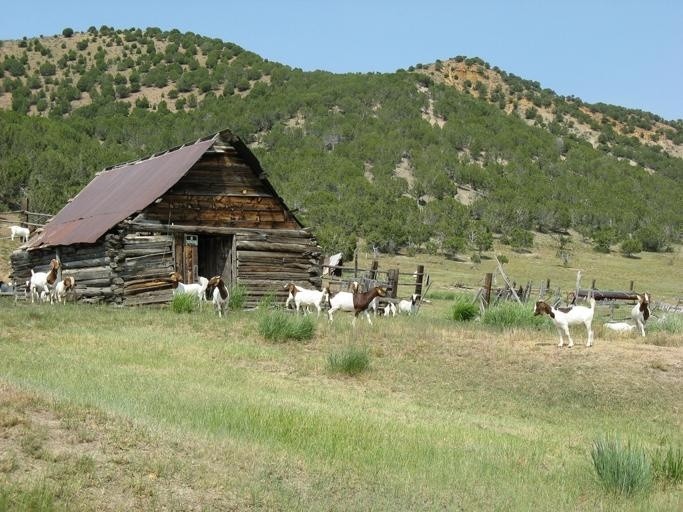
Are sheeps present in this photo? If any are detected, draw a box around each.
[24,259,77,306]
[534,298,596,348]
[169,272,207,300]
[603,323,636,333]
[283,281,422,328]
[7,226,30,243]
[198,276,209,303]
[631,292,652,337]
[208,275,229,318]
